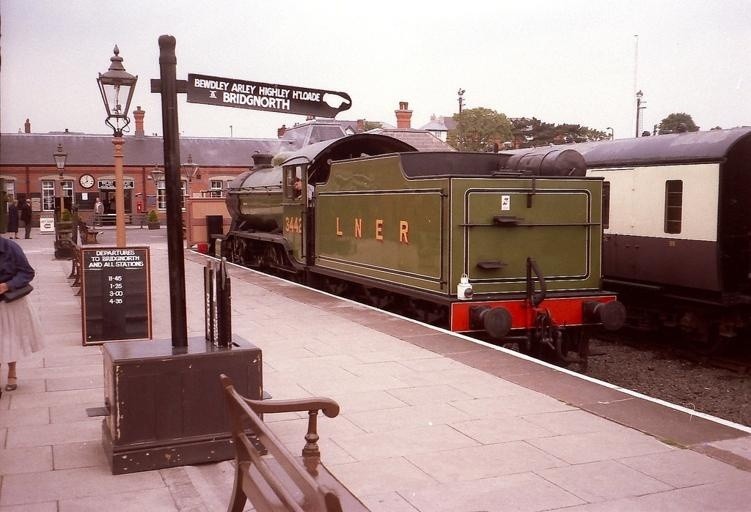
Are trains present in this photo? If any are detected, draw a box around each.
[218,114,629,377]
[478,125,750,353]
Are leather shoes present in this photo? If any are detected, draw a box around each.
[7,377,16,390]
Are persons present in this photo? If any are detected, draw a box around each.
[7,199,20,239]
[94,197,104,226]
[290,177,315,207]
[0,238,35,391]
[21,199,32,239]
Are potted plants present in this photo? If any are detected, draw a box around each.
[148,210,161,230]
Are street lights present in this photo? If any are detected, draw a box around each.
[456,88,468,152]
[95,44,138,246]
[182,153,200,199]
[606,126,615,140]
[52,142,71,222]
[635,91,648,138]
[150,163,164,211]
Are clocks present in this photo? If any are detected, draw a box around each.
[79,174,96,189]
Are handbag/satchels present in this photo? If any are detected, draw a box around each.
[3,284,33,302]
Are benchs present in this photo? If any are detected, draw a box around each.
[215,371,346,511]
[66,211,101,297]
